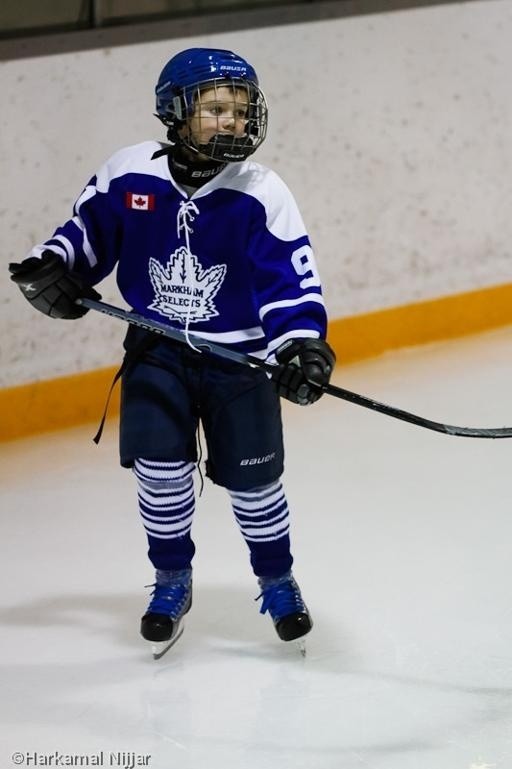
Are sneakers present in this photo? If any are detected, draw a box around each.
[141,569,192,641]
[255,569,312,641]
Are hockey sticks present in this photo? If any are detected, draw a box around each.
[71,296,512,438]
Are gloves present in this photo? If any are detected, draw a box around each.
[11,249,102,318]
[266,338,336,406]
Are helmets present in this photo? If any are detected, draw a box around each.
[156,49,267,163]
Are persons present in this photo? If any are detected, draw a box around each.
[13,47,336,642]
